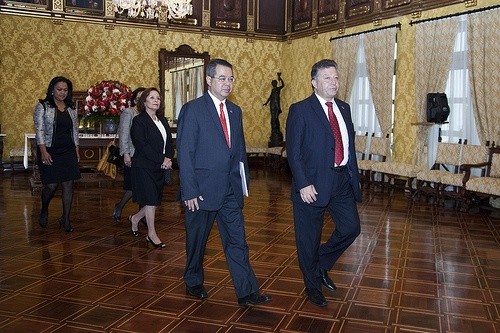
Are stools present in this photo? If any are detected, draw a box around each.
[9,149,32,172]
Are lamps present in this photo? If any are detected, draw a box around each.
[112,0,194,20]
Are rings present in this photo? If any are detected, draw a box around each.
[42,161,45,163]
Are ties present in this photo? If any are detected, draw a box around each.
[220,102,230,145]
[326,101,344,167]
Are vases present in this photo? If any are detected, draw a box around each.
[105,119,118,134]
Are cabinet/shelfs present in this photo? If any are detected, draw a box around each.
[23,133,124,192]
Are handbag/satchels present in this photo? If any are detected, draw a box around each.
[107,144,130,170]
[162,168,173,186]
[98,141,117,179]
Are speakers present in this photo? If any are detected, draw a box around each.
[427,93,450,123]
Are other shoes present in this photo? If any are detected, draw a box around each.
[113,202,122,223]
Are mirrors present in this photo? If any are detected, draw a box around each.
[158,44,211,133]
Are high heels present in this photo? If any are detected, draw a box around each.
[127,214,139,237]
[39,208,48,227]
[146,235,166,248]
[58,218,75,231]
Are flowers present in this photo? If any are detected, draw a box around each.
[81,80,134,118]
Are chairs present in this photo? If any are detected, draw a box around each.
[246,132,500,214]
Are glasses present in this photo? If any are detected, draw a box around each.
[212,77,235,83]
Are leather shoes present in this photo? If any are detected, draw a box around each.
[320,265,336,290]
[186,282,208,298]
[306,286,327,307]
[238,291,272,306]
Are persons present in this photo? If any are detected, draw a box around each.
[285,60,361,306]
[113,87,174,247]
[263,76,284,133]
[34,76,82,233]
[177,59,273,307]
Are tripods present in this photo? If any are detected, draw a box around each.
[410,127,448,201]
